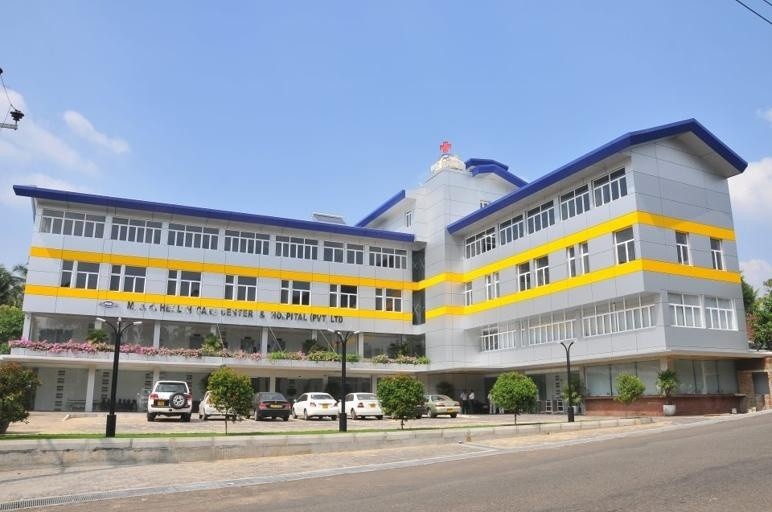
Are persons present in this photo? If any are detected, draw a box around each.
[469,389,475,414]
[487,390,496,415]
[498,406,505,415]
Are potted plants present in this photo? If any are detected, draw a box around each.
[657,373,681,414]
[561,380,582,413]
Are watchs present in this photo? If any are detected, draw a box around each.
[460,389,469,414]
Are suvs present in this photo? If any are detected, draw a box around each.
[147,380,193,422]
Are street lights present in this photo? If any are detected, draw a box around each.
[554,336,582,426]
[324,327,362,431]
[92,314,142,439]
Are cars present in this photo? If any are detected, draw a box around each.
[290,391,341,420]
[337,392,387,421]
[246,391,293,422]
[416,394,462,419]
[198,389,238,422]
